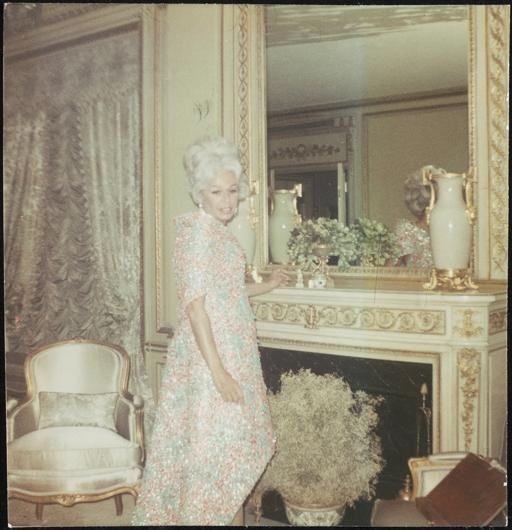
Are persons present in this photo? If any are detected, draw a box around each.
[132,138,291,525]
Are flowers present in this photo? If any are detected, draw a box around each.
[287,217,433,267]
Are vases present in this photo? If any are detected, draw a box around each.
[423,167,479,292]
[268,190,302,264]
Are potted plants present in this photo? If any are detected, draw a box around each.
[249,369,387,528]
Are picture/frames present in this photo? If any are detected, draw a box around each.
[3,2,159,394]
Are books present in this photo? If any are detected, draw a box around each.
[416,453,507,528]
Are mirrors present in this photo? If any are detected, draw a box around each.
[223,2,508,288]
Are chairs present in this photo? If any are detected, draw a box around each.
[4,339,147,522]
[370,454,510,529]
